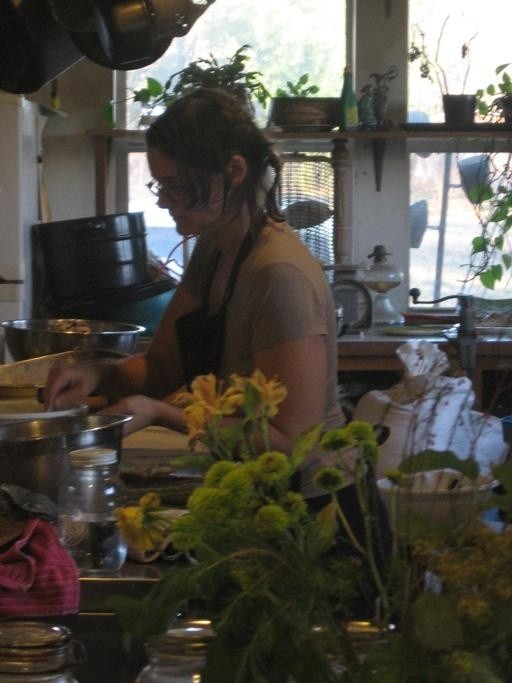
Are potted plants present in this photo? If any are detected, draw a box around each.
[407,11,476,127]
[458,61,510,288]
[95,40,345,132]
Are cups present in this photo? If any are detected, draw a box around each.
[461,154,493,202]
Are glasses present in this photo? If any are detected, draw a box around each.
[145,180,191,197]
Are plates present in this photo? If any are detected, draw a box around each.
[378,324,451,337]
[1,316,146,362]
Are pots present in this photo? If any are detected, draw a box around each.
[95,0,155,63]
[31,210,148,293]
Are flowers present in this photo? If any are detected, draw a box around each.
[91,364,512,683]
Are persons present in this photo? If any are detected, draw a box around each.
[41,89,392,621]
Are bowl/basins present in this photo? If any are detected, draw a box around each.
[0,397,89,427]
[372,464,501,522]
[0,349,134,398]
[1,414,137,503]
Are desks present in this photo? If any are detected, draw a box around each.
[85,322,511,412]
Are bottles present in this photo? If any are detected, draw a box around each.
[362,245,407,326]
[57,446,130,575]
[0,620,84,683]
[134,627,219,683]
[340,65,359,130]
